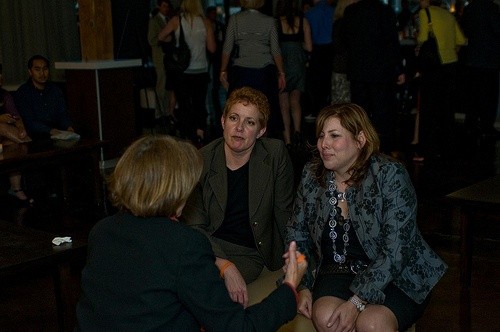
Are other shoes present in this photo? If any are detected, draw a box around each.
[14,213,23,226]
[294,131,303,152]
[285,144,294,154]
[62,190,72,206]
[8,188,28,201]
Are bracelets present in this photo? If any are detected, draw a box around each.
[220,71,227,74]
[220,261,236,277]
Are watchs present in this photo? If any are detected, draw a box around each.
[348,297,366,313]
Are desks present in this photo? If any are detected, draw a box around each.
[0,220,88,332]
[54,57,143,178]
[0,133,111,228]
[446,175,500,332]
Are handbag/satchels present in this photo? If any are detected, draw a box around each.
[415,7,442,82]
[163,15,191,81]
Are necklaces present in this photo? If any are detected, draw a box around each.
[329,171,351,270]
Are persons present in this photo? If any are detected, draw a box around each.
[0,0,500,332]
